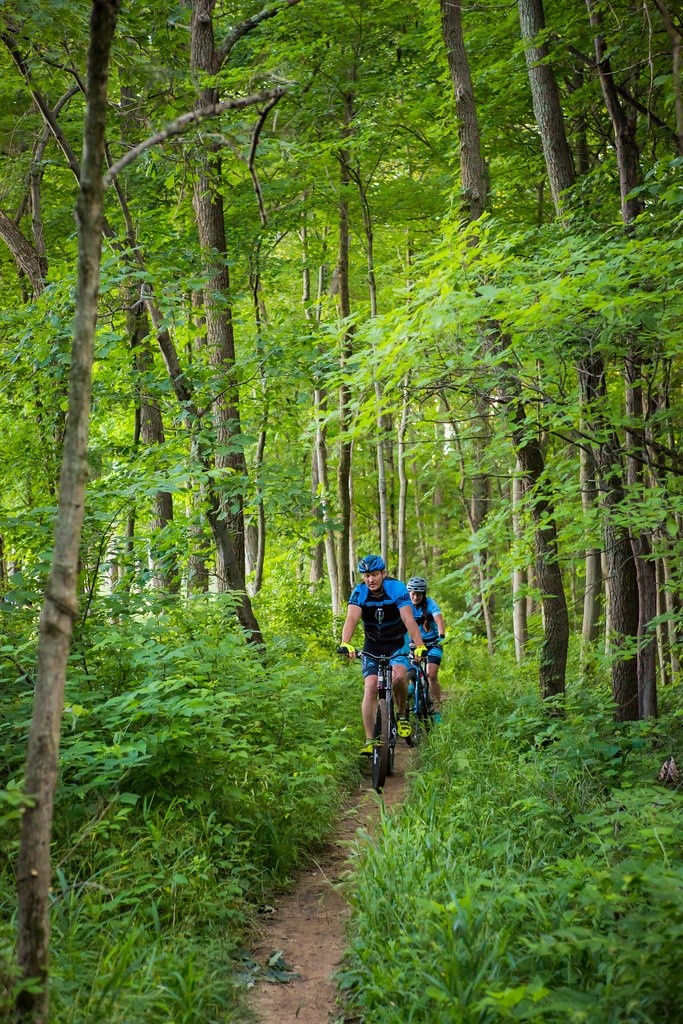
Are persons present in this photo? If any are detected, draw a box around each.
[337,555,428,757]
[407,578,445,726]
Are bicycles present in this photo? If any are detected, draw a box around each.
[395,635,450,748]
[336,648,428,792]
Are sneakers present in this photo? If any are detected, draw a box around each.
[358,743,374,758]
[434,712,443,723]
[395,711,412,738]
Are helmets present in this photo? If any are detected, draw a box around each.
[407,576,428,592]
[358,554,385,572]
